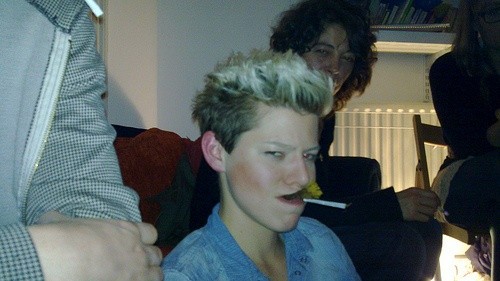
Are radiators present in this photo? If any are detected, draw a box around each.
[328,107,447,197]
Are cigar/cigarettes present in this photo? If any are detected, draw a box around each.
[303,198,347,210]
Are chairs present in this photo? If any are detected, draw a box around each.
[412,114,500,280]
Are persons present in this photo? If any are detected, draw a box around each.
[164,49,360,280]
[0,0,165,281]
[190,0,443,281]
[428,1,500,281]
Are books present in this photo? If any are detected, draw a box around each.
[369,0,458,25]
